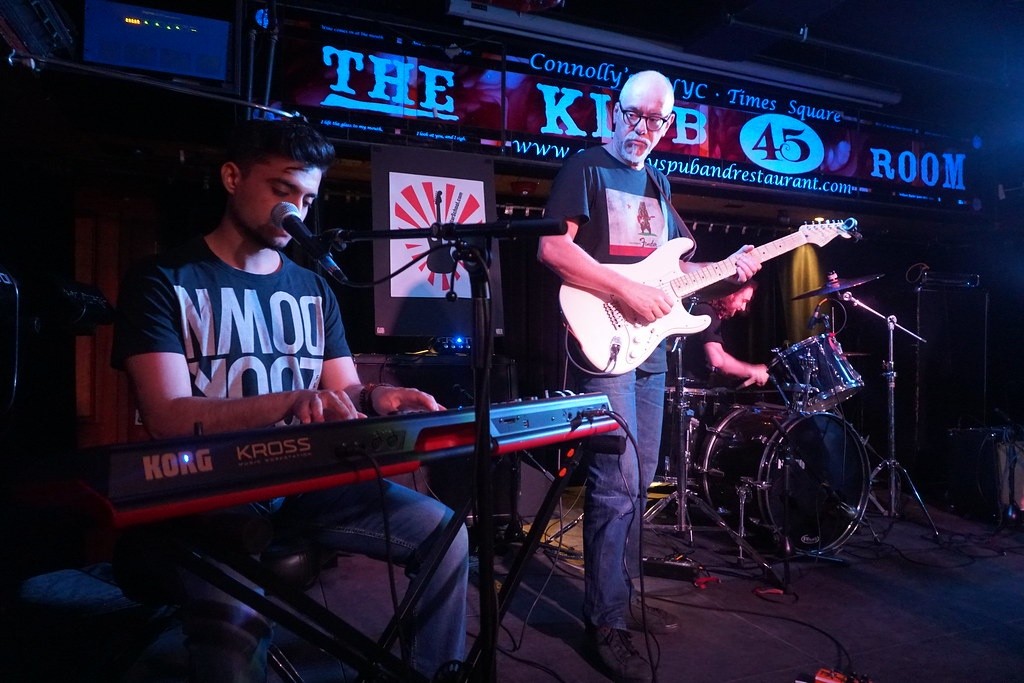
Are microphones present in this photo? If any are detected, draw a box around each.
[451,383,474,403]
[271,201,349,287]
[808,304,821,329]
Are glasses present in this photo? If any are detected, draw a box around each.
[618,101,670,131]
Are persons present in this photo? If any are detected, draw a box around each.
[683,278,770,391]
[108,121,472,682]
[536,69,762,682]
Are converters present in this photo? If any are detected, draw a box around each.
[642,556,699,581]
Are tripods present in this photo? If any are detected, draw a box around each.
[840,291,945,546]
[641,296,799,602]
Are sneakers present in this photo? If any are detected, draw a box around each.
[584,621,652,683]
[624,598,680,635]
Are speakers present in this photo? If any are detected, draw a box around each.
[915,279,1024,524]
[352,352,520,525]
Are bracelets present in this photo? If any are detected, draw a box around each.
[358,382,394,417]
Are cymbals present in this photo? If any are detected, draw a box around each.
[791,271,885,303]
[844,352,870,360]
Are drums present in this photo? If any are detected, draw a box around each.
[663,387,728,418]
[766,331,864,412]
[703,402,872,558]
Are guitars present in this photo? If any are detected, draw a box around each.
[559,218,862,379]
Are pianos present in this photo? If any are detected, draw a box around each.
[83,389,621,530]
